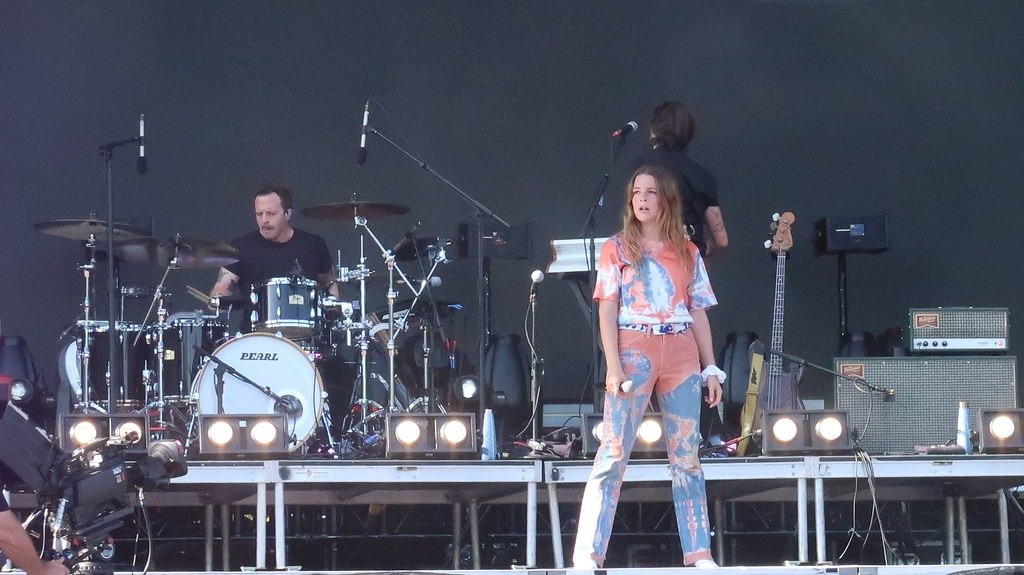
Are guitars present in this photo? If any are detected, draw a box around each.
[740,210,809,453]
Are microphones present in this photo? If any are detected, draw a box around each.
[281,399,301,413]
[604,381,635,393]
[529,269,544,301]
[397,277,442,287]
[613,121,638,136]
[136,113,146,174]
[357,99,370,165]
[394,220,423,251]
[884,389,895,402]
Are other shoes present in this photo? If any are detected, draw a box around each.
[695,559,719,567]
[574,560,598,569]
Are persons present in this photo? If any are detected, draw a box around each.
[209,185,347,442]
[643,102,729,447]
[572,166,723,569]
[0,487,71,575]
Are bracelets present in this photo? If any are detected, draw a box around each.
[701,365,726,388]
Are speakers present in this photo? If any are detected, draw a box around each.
[814,214,891,257]
[833,354,1016,454]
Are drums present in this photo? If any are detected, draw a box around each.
[294,320,339,360]
[143,321,230,402]
[250,275,323,341]
[75,319,151,411]
[189,331,326,453]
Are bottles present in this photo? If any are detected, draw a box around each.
[481,408,498,460]
[956,401,973,454]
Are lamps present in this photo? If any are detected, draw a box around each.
[760,409,855,456]
[976,407,1024,453]
[60,413,152,463]
[198,414,288,459]
[580,413,668,460]
[386,413,478,460]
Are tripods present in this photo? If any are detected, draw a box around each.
[339,206,448,460]
[72,223,197,441]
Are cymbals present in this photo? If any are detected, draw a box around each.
[394,236,440,262]
[112,235,242,269]
[33,217,148,243]
[300,201,411,221]
